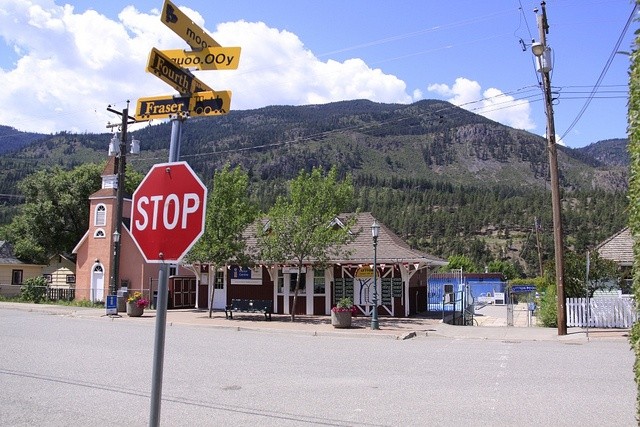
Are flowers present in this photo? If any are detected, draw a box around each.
[127,291,150,308]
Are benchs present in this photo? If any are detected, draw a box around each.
[224,298,274,320]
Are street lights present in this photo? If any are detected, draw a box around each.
[112,227,120,297]
[532,42,570,336]
[370,219,382,329]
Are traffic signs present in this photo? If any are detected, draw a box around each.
[146,47,214,93]
[158,46,241,69]
[160,0,222,47]
[135,89,232,121]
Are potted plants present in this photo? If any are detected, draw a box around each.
[331,296,356,328]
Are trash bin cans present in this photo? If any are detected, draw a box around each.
[153,289,171,310]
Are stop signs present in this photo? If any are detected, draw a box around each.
[130,160,207,263]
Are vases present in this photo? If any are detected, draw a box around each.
[125,302,144,316]
